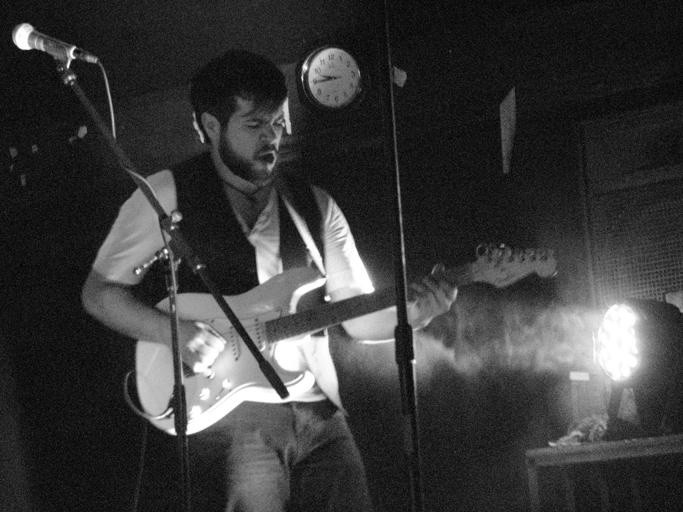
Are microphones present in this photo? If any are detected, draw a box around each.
[12,22,96,68]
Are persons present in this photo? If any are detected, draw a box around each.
[80,49,459,509]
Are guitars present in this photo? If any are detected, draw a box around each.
[134,245,558,439]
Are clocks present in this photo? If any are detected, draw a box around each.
[296,47,364,111]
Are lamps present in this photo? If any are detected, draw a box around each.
[592,296,681,440]
